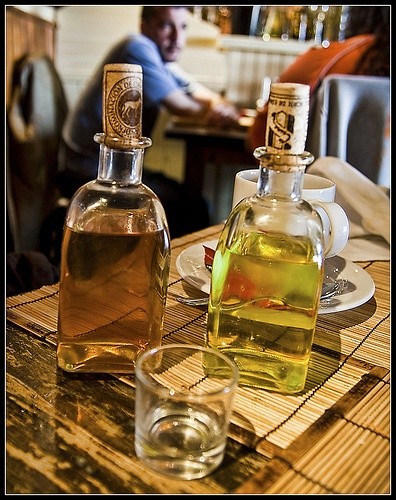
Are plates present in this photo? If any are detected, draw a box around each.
[174,240,378,314]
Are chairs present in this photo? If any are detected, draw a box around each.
[6,53,72,262]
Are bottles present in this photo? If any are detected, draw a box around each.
[54,63,171,374]
[187,7,342,48]
[202,81,323,395]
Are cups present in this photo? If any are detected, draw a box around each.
[134,341,240,481]
[233,167,350,262]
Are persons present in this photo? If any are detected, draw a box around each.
[57,4,238,240]
[249,5,390,152]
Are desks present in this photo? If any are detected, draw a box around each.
[162,106,257,206]
[6,215,390,494]
